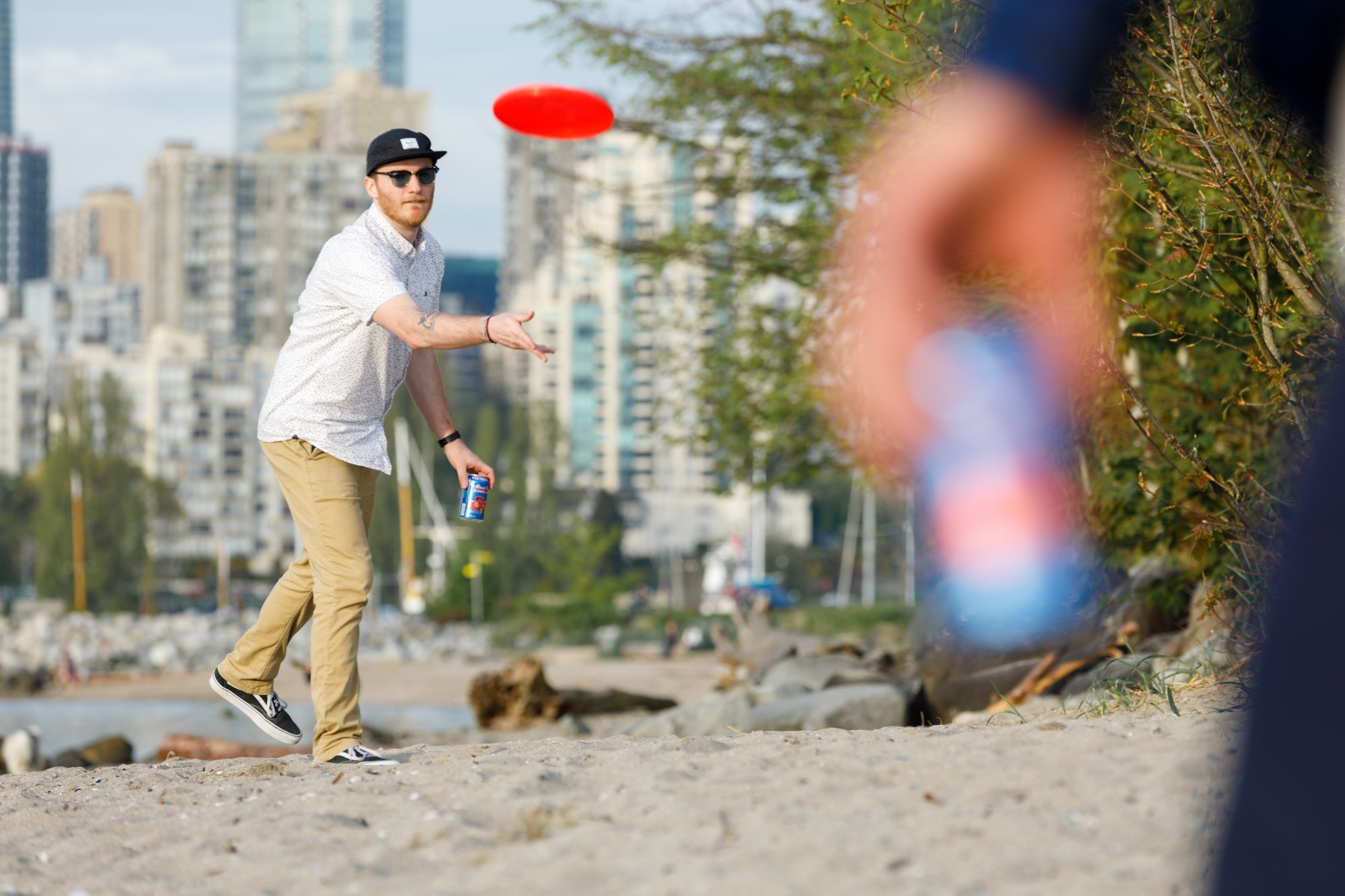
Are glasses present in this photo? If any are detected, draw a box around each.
[373,165,439,187]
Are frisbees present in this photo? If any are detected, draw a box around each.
[494,86,613,141]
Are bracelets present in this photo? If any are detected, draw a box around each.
[481,315,496,344]
[438,428,462,450]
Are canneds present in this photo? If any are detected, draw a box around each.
[908,318,1116,650]
[458,474,490,522]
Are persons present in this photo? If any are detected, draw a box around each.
[1,532,790,773]
[820,0,1345,895]
[209,128,556,773]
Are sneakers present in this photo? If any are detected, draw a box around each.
[327,746,401,766]
[209,667,302,745]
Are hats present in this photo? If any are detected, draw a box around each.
[366,128,447,176]
